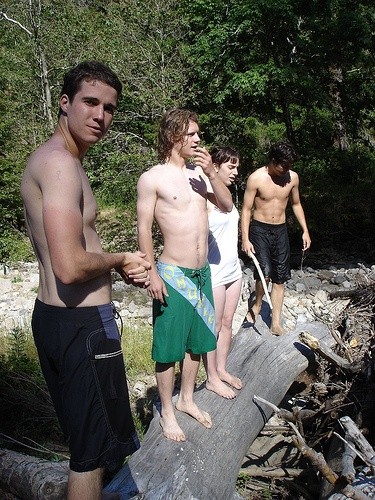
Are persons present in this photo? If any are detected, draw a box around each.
[20,61,151,500]
[241,141,310,336]
[136,110,233,441]
[203,144,243,400]
